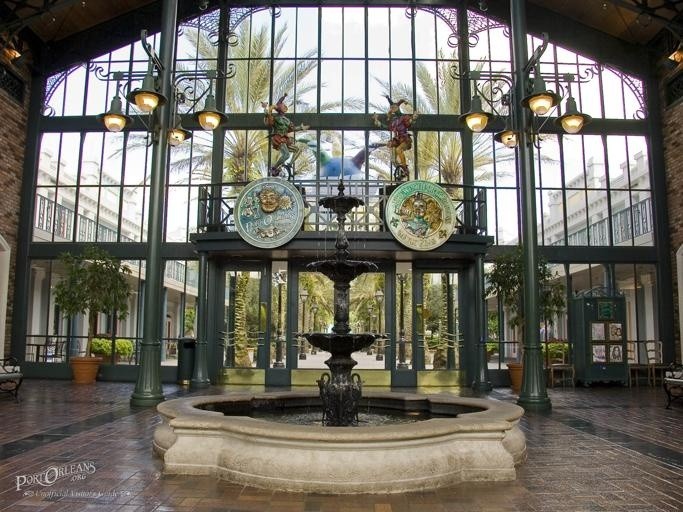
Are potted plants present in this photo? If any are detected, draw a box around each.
[91,338,133,364]
[52,242,131,384]
[482,245,565,394]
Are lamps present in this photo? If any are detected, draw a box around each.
[167,110,191,146]
[552,83,593,134]
[458,80,494,132]
[125,56,168,114]
[96,78,135,134]
[192,79,229,132]
[520,60,562,115]
[493,105,520,148]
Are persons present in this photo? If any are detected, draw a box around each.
[402,193,430,237]
[611,325,621,340]
[259,189,278,213]
[612,346,621,360]
[261,93,302,177]
[371,96,419,176]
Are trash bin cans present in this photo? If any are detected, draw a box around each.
[176,337,196,385]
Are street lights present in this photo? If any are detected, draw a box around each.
[446,30,595,412]
[298,289,328,361]
[396,272,408,369]
[351,289,383,362]
[272,272,285,369]
[92,28,236,413]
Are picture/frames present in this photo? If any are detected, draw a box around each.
[589,319,608,343]
[609,343,626,365]
[606,322,624,343]
[590,343,608,363]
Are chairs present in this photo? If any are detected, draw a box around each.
[663,361,683,410]
[644,340,670,388]
[0,356,24,404]
[546,337,575,390]
[627,341,641,390]
